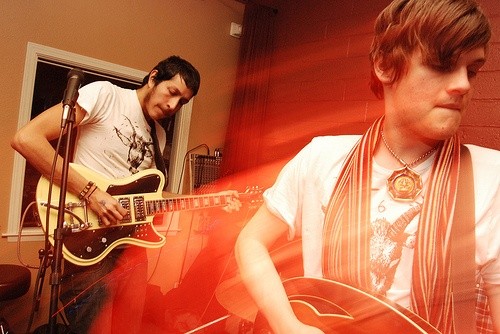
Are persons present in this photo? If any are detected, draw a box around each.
[10,54,242,334]
[235,0,499,333]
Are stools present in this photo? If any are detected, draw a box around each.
[0,264,31,334]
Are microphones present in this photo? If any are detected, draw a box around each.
[61,69,85,128]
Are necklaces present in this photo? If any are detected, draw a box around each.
[379,127,441,202]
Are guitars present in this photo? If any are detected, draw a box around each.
[35,163,269,267]
[252,277,443,334]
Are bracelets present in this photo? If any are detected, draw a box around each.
[78,180,98,201]
